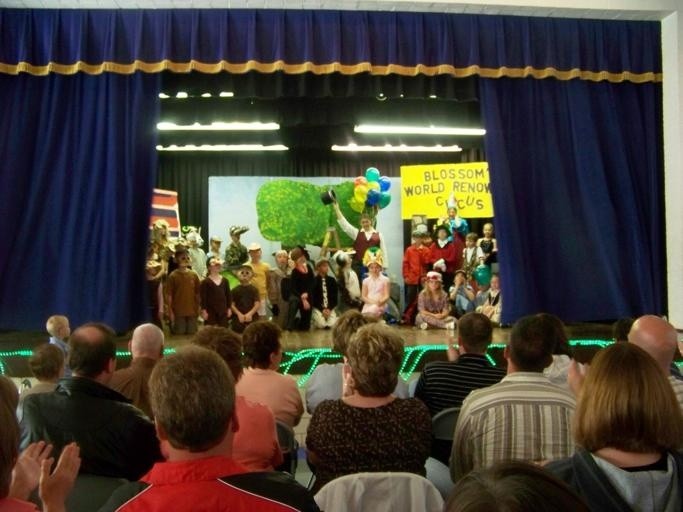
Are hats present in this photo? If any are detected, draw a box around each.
[446,191,459,211]
[432,223,452,239]
[361,246,385,268]
[271,248,289,257]
[241,260,256,274]
[314,255,330,270]
[411,223,429,236]
[288,246,306,261]
[319,186,338,206]
[421,270,444,284]
[210,233,225,242]
[455,269,467,275]
[248,241,262,252]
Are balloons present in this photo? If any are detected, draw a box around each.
[349,167,392,213]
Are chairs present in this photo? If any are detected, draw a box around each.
[276,421,299,476]
[431,407,461,463]
[29,475,131,510]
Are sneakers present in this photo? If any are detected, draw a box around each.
[418,322,429,331]
[444,320,457,331]
